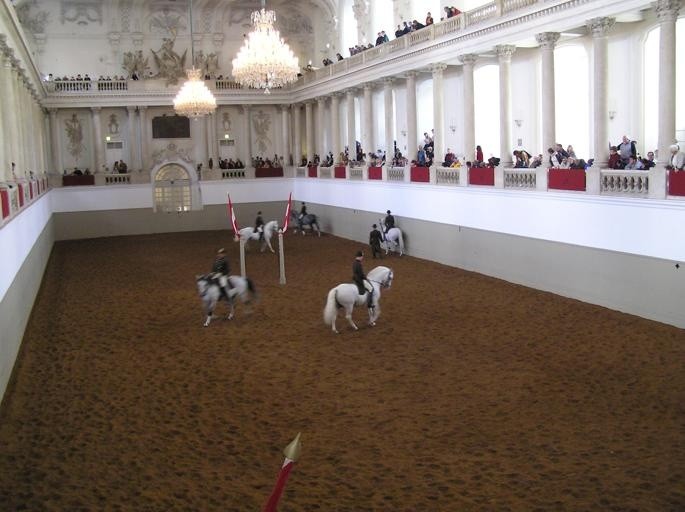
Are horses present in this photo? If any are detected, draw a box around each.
[195,272,259,327]
[323,265,394,334]
[290,208,321,238]
[232,220,279,253]
[378,218,405,256]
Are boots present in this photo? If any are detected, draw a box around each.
[367,292,376,308]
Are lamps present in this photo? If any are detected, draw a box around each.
[170,0,304,123]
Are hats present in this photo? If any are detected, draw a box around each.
[370,223,378,227]
[353,248,365,257]
[386,209,392,213]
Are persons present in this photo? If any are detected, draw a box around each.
[196,153,293,177]
[297,42,374,79]
[62,159,128,183]
[505,133,683,189]
[375,6,463,46]
[44,70,253,91]
[252,209,264,243]
[382,211,394,235]
[350,250,375,309]
[297,201,306,223]
[213,247,231,304]
[297,128,502,170]
[367,223,384,260]
